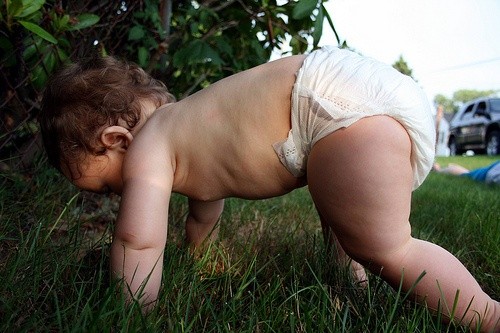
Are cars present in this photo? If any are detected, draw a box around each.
[446,96,500,157]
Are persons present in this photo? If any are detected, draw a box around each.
[431,159,500,186]
[41,45,500,333]
